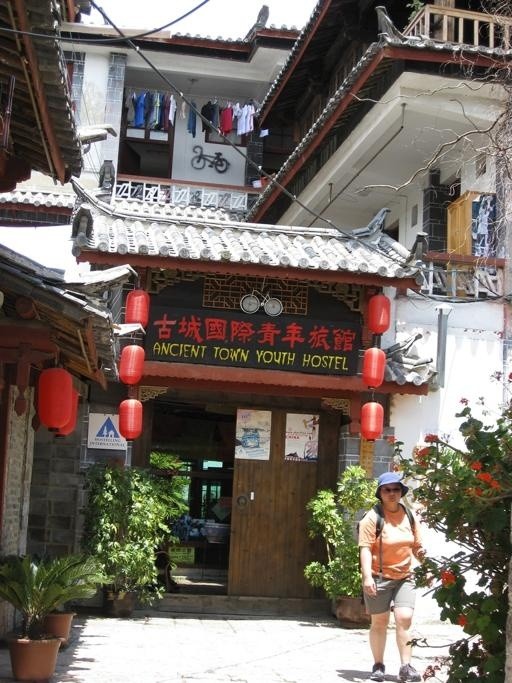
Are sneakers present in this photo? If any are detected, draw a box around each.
[398,663,420,681]
[370,663,385,681]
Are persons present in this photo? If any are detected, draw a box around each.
[356,470,435,683]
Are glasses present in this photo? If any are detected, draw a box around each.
[381,487,402,494]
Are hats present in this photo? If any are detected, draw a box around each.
[375,472,409,499]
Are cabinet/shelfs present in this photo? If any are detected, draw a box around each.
[446,189,496,298]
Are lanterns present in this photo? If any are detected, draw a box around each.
[37,364,74,433]
[117,394,146,442]
[122,288,150,329]
[358,343,389,390]
[357,398,386,444]
[364,291,393,336]
[117,344,146,388]
[54,386,81,442]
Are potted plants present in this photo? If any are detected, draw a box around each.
[80,451,194,620]
[303,462,382,630]
[1,547,112,682]
